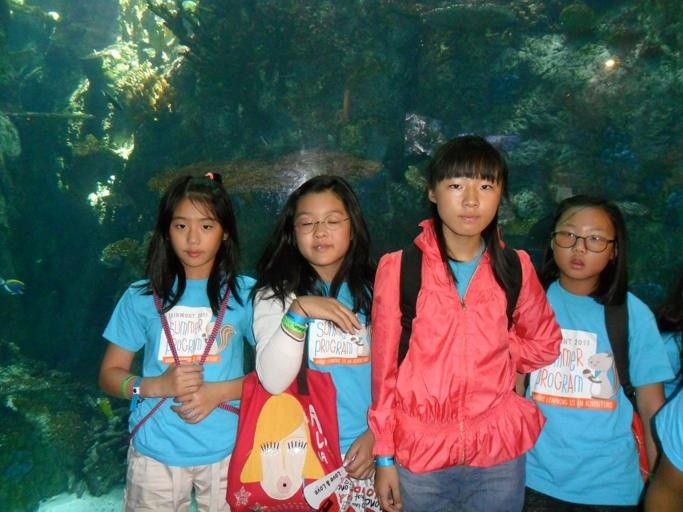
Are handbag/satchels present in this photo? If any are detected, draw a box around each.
[227,369,344,512]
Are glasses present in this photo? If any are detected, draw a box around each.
[295,215,350,234]
[551,231,613,252]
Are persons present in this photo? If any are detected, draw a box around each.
[367,134,562,512]
[97,173,258,512]
[247,175,380,512]
[522,195,676,512]
[643,388,683,512]
[655,290,683,400]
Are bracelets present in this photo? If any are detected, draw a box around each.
[121,374,136,400]
[281,299,309,341]
[129,377,143,412]
[375,456,395,466]
